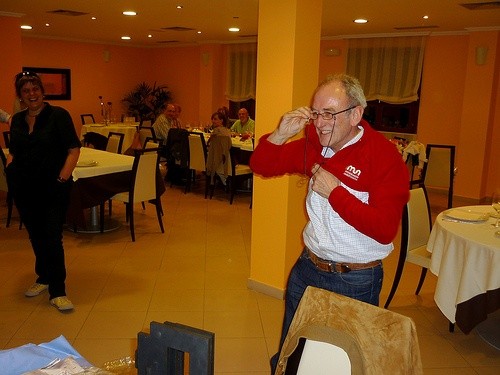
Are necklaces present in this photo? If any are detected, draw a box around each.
[27,110,38,117]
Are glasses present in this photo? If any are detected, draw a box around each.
[309,105,356,120]
[16,72,39,84]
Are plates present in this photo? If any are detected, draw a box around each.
[74,159,99,168]
[443,207,490,222]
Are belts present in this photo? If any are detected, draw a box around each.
[304,246,380,273]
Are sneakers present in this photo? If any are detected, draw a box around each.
[24,284,49,296]
[49,295,75,311]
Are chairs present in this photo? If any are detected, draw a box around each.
[105,131,126,154]
[121,114,137,122]
[186,132,217,199]
[139,126,166,160]
[273,286,415,374]
[121,137,165,216]
[411,144,457,209]
[95,148,166,242]
[167,127,195,194]
[0,146,24,230]
[81,114,96,147]
[209,134,253,208]
[138,120,153,130]
[383,187,457,334]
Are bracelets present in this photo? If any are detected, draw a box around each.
[57,175,67,183]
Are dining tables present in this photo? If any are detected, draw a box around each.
[186,129,254,154]
[435,205,500,331]
[387,138,413,188]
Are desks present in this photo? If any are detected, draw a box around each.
[1,147,135,234]
[82,122,140,152]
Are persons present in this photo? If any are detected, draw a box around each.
[208,111,229,135]
[171,104,182,129]
[152,104,184,189]
[230,107,254,164]
[249,74,411,375]
[5,70,82,311]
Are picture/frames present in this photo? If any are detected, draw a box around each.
[22,66,72,101]
